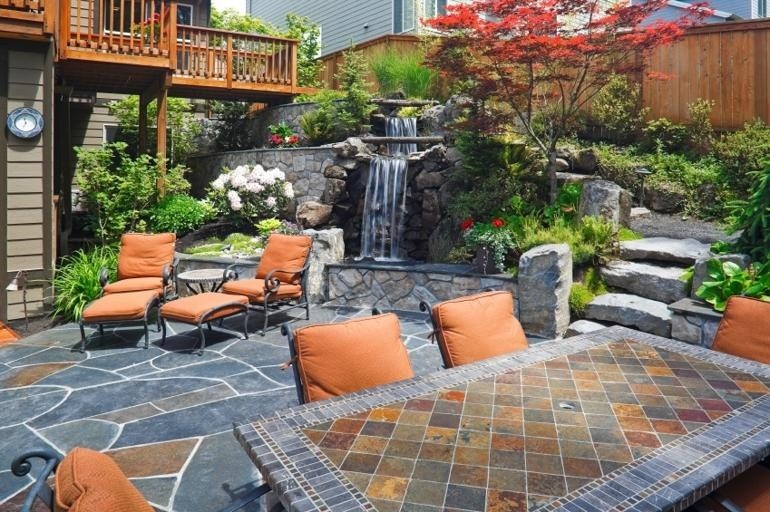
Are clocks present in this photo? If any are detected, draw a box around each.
[5,107,46,142]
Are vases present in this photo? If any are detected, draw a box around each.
[474,241,494,274]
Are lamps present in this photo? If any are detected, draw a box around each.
[6,269,32,333]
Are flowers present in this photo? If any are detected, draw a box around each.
[266,123,304,149]
[457,214,512,278]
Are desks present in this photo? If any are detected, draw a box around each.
[175,268,236,330]
[233,321,768,512]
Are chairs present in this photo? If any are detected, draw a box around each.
[219,232,314,335]
[99,230,178,297]
[713,292,769,511]
[419,285,533,370]
[9,445,160,512]
[276,306,414,407]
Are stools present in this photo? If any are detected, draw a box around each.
[76,290,161,351]
[157,290,251,356]
